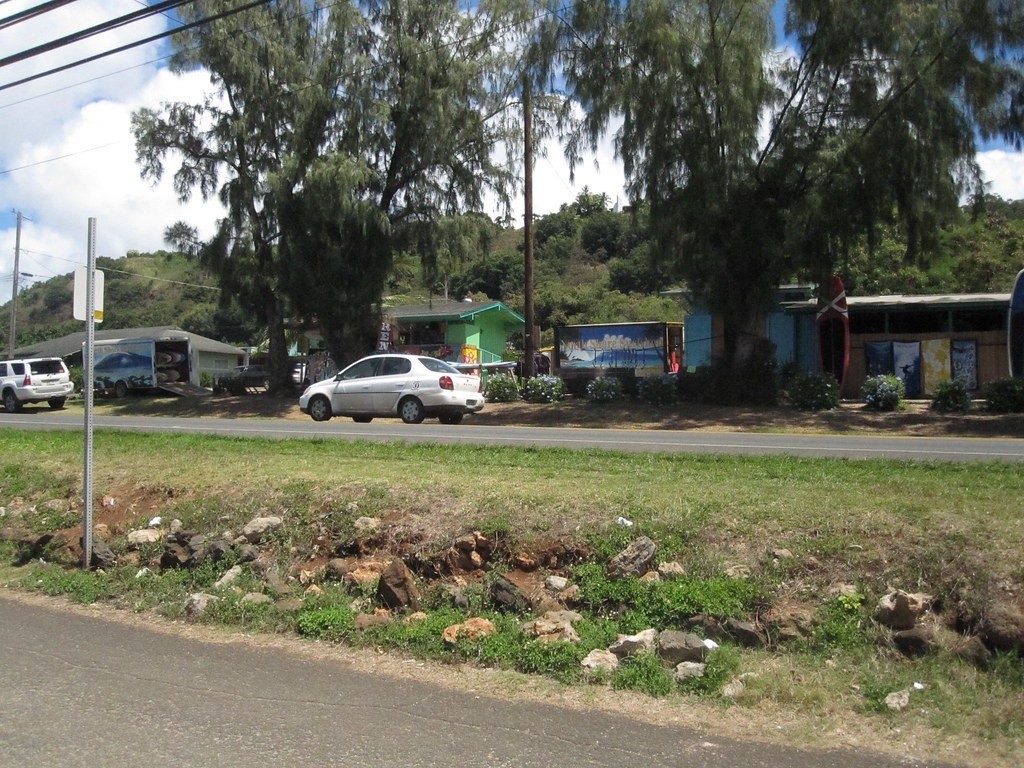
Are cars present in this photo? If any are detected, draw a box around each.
[293,362,306,384]
[298,353,487,426]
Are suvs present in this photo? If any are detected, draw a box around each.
[0,358,75,413]
[230,365,273,390]
